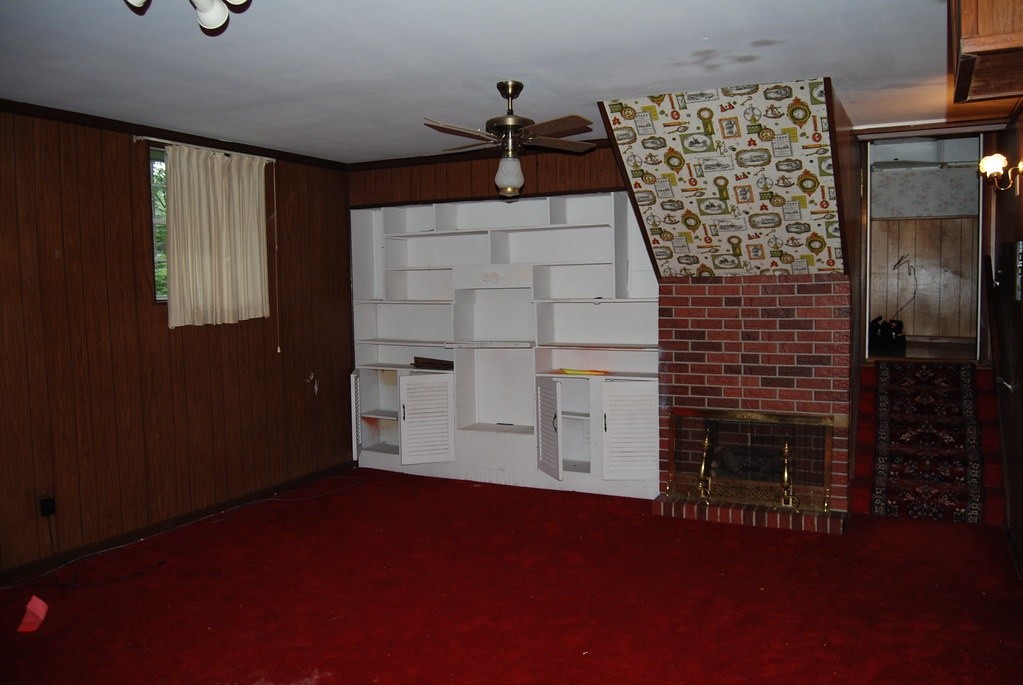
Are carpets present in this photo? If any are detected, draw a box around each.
[875,359,985,525]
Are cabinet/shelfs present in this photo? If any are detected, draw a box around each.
[350,191,659,499]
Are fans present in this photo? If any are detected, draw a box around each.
[423,80,596,153]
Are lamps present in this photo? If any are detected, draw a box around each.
[979,154,1023,191]
[191,0,228,29]
[226,0,247,5]
[127,0,147,7]
[495,139,524,197]
